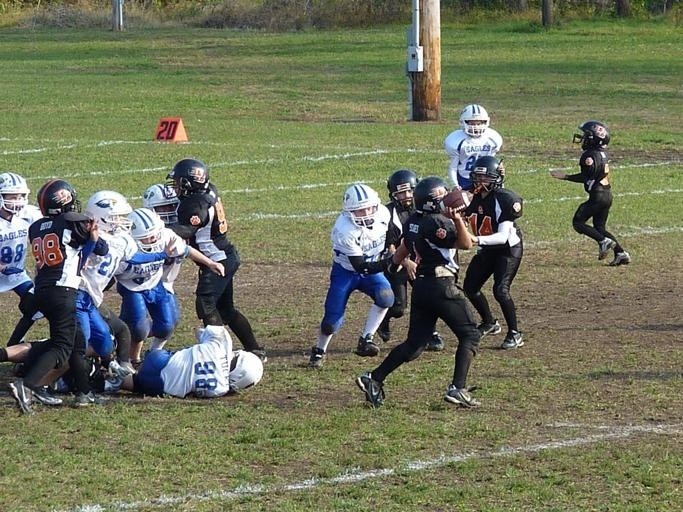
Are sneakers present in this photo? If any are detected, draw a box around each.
[444,384,481,408]
[609,250,631,266]
[249,347,267,363]
[425,331,444,351]
[597,236,616,260]
[8,358,136,415]
[308,346,326,371]
[477,318,502,338]
[500,329,524,350]
[377,318,390,343]
[354,370,385,409]
[356,332,381,356]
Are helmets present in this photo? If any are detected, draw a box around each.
[571,120,610,152]
[412,177,447,216]
[469,155,505,194]
[342,184,381,228]
[0,158,211,255]
[387,169,419,213]
[458,103,491,138]
[229,349,263,394]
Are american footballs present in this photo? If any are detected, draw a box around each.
[437,190,474,213]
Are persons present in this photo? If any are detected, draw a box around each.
[442,102,502,195]
[306,182,398,373]
[462,156,525,350]
[369,168,447,352]
[354,172,486,408]
[547,120,631,267]
[0,159,268,415]
